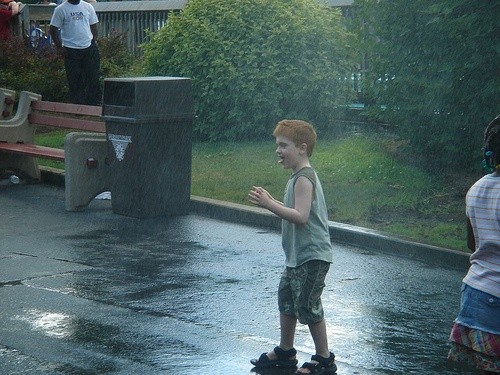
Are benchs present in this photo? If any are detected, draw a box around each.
[0,91,110,213]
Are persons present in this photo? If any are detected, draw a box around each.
[247,120,338,375]
[48,0,100,106]
[457,114,500,375]
[0,0,24,43]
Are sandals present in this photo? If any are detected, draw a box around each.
[250,345,298,368]
[296,351,338,375]
